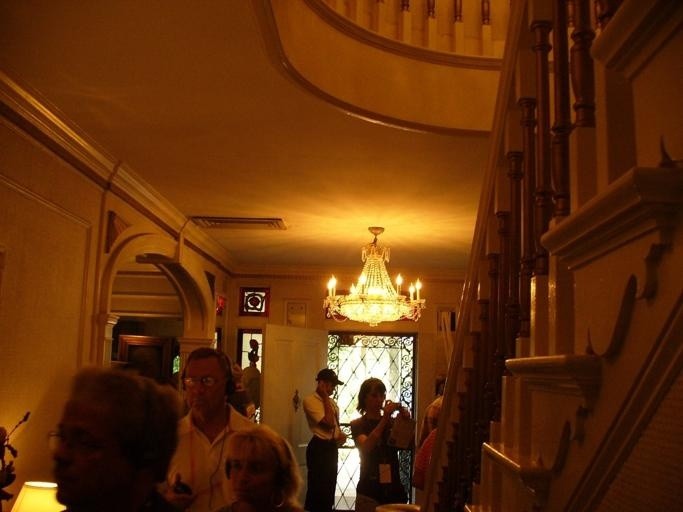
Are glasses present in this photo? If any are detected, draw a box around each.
[182,375,219,388]
[47,430,107,465]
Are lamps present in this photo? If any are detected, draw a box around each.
[9,480,68,512]
[321,228,426,328]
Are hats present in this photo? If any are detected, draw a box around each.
[315,368,343,386]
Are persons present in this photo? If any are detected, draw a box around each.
[349,377,417,512]
[418,393,444,446]
[211,424,305,512]
[303,369,347,512]
[155,348,253,512]
[52,365,180,511]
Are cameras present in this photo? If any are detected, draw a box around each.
[389,402,402,409]
[174,479,192,496]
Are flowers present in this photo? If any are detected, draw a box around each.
[0,411,30,512]
